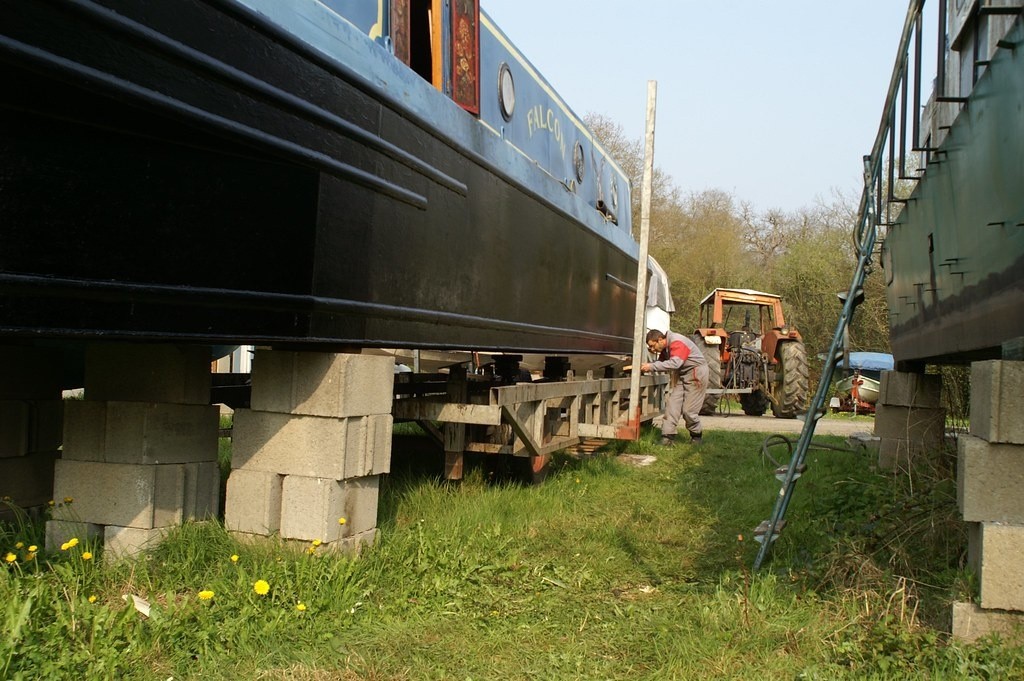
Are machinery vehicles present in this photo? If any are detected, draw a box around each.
[695,287,809,419]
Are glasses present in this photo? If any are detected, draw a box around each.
[648,342,658,349]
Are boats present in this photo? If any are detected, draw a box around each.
[837,369,880,404]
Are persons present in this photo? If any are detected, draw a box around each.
[641,330,710,446]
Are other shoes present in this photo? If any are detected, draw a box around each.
[690,432,703,444]
[662,435,675,446]
